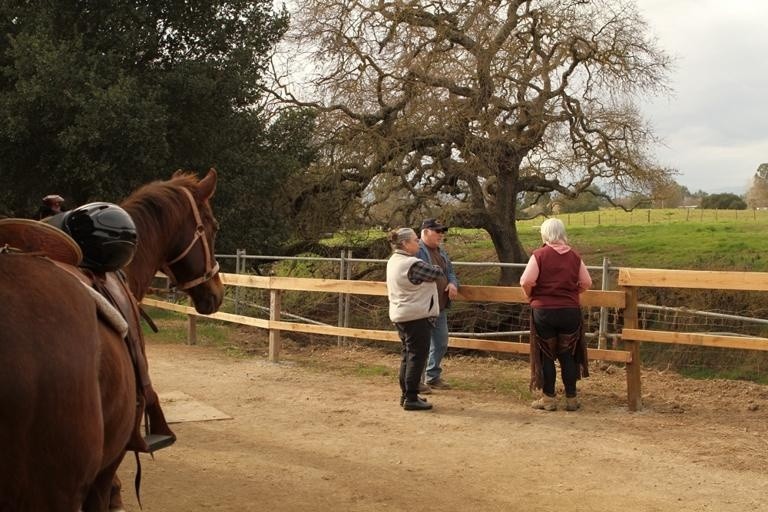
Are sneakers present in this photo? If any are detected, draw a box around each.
[567,398,580,411]
[400,396,427,407]
[531,397,556,411]
[404,398,433,410]
[419,383,432,394]
[426,376,450,390]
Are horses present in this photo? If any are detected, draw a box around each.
[0,167,226,512]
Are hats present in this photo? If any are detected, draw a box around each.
[42,195,64,204]
[422,218,448,231]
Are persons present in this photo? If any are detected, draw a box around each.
[32,195,64,222]
[520,218,593,411]
[415,218,460,395]
[385,227,444,411]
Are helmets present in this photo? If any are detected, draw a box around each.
[61,202,138,272]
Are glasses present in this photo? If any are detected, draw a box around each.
[436,230,444,234]
